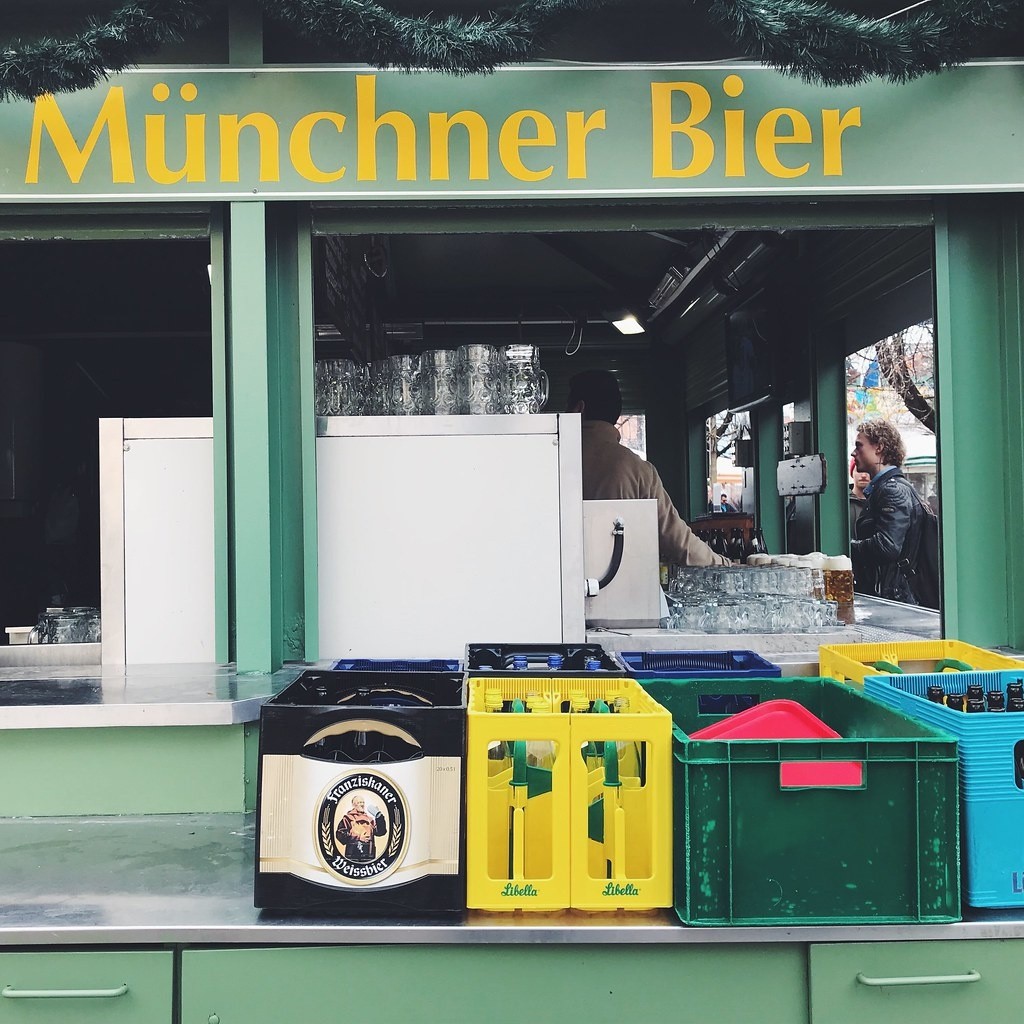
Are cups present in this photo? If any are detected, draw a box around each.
[665,564,837,634]
[26,606,102,645]
[313,343,549,417]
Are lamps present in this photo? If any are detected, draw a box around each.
[611,306,646,336]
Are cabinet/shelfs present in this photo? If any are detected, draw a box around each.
[0,938,1024,1024]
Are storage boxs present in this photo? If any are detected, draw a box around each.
[255,639,1024,923]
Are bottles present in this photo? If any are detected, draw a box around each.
[747,552,855,607]
[309,683,380,764]
[478,652,643,790]
[928,682,1024,790]
[696,527,770,565]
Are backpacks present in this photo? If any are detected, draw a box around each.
[871,478,940,610]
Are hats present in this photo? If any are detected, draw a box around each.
[850,458,856,478]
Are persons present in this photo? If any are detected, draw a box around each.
[567,369,735,566]
[708,486,713,512]
[852,421,922,605]
[849,458,870,540]
[721,494,737,512]
